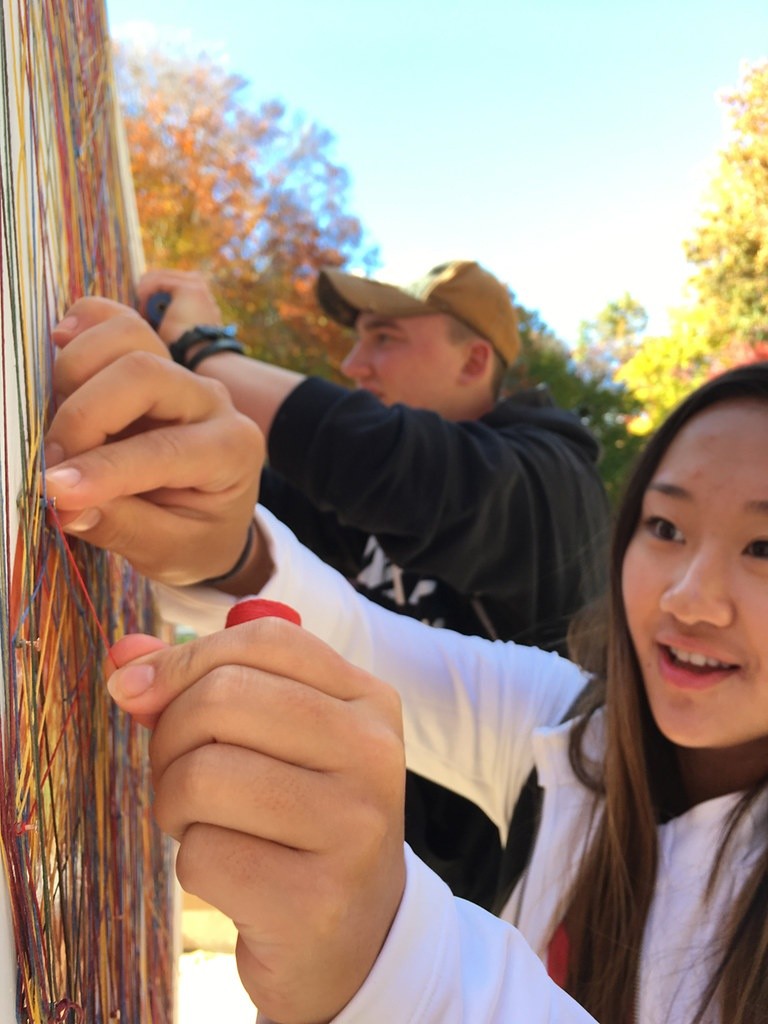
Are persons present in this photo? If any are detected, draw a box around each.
[139,260,618,917]
[34,298,767,1024]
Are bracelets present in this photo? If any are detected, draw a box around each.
[171,320,244,371]
[206,525,254,584]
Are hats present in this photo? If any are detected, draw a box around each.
[315,259,521,369]
